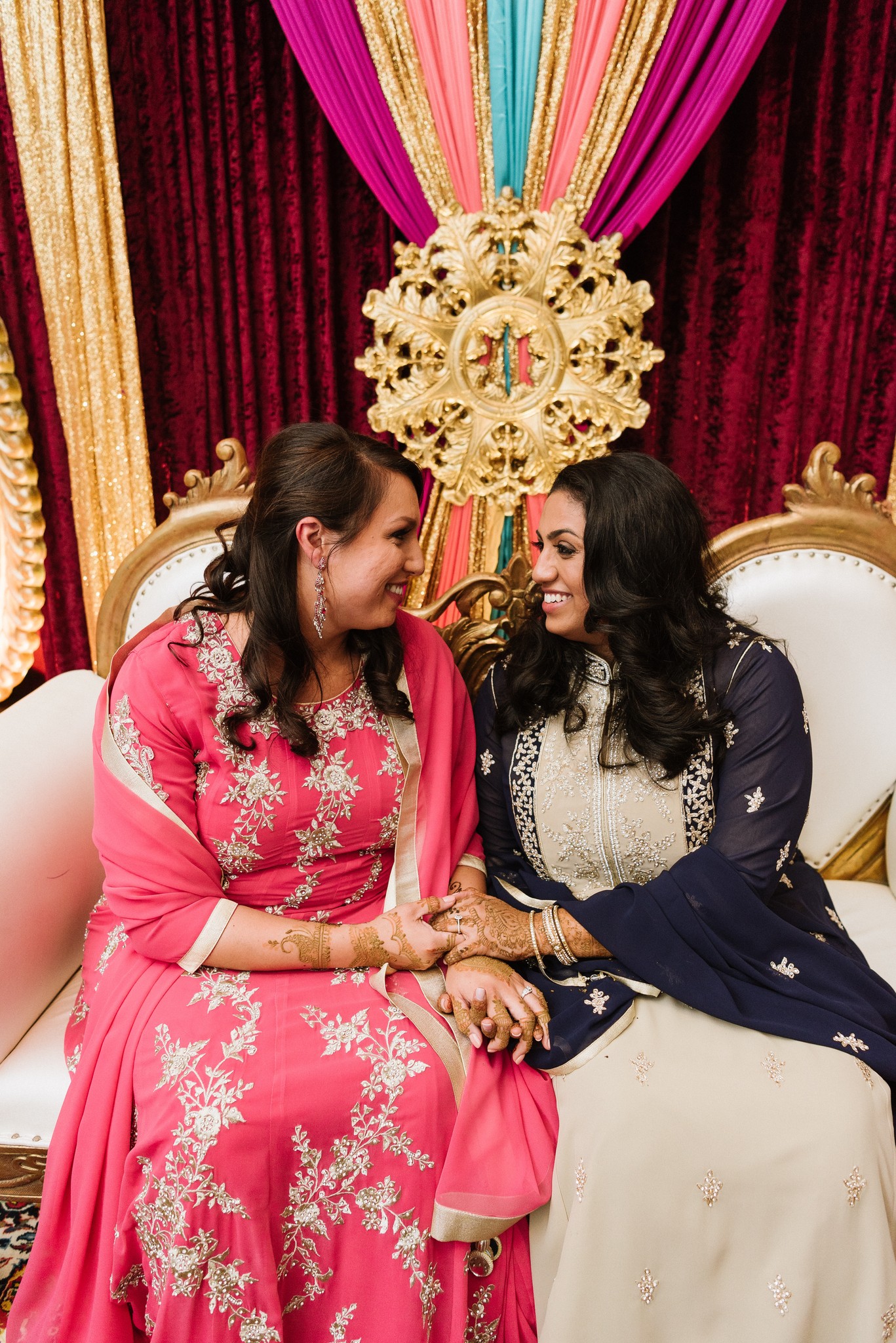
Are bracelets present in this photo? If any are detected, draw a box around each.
[553,905,579,963]
[542,905,575,966]
[528,909,548,969]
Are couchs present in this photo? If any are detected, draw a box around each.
[0,440,896,1183]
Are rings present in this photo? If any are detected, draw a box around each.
[453,914,463,935]
[521,985,534,1000]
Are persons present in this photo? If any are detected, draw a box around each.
[34,416,559,1342]
[433,446,895,1343]
